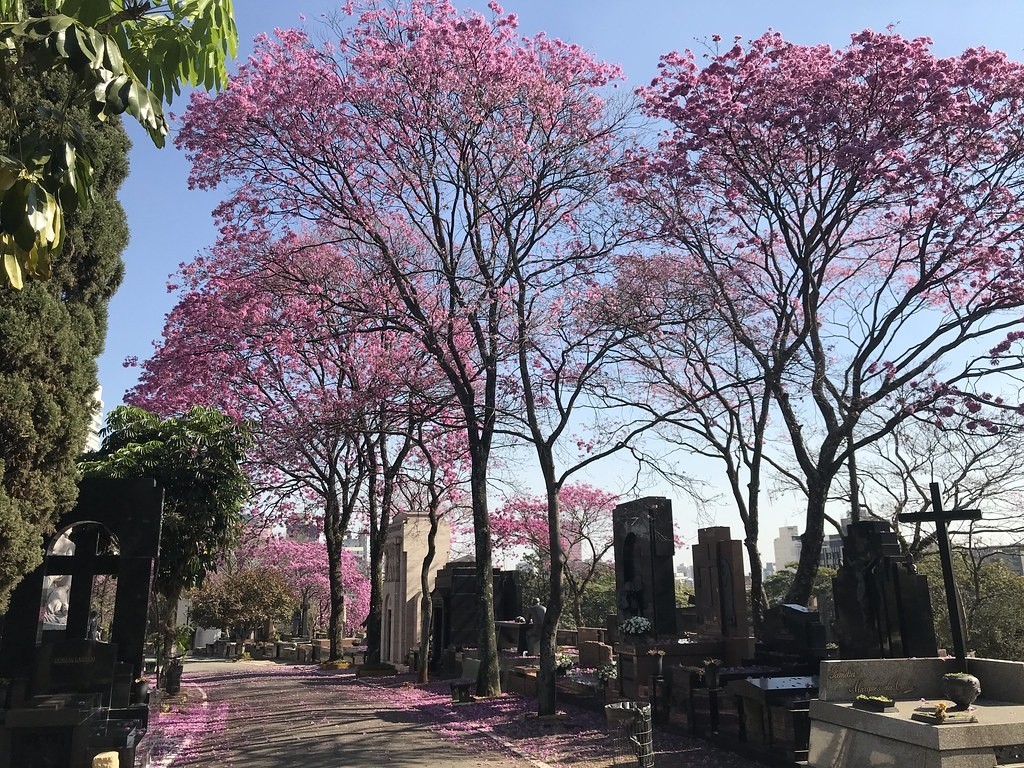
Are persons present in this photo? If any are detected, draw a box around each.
[88,611,103,638]
[525,597,546,656]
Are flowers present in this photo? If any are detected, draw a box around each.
[532,652,618,689]
[919,696,948,724]
[619,616,653,636]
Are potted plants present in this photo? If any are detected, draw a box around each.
[852,693,895,713]
[148,623,195,696]
